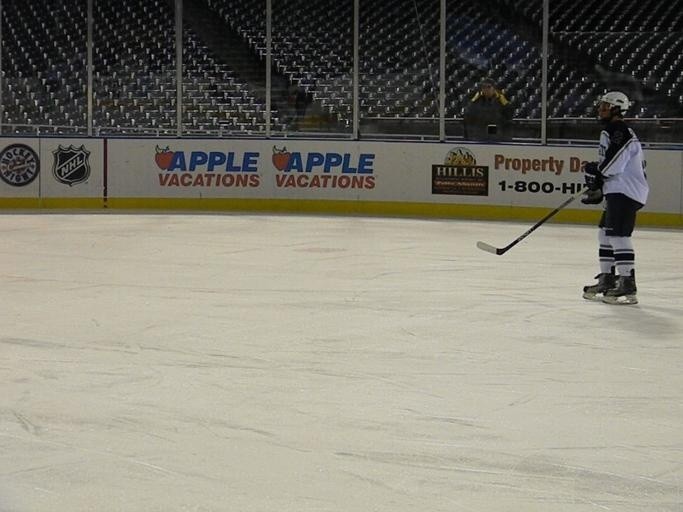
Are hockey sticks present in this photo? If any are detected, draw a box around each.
[476,186,588,256]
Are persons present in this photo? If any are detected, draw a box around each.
[584,91,649,298]
[462,78,515,139]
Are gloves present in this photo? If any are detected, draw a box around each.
[581,161,609,205]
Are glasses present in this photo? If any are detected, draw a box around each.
[481,85,492,89]
[599,101,616,112]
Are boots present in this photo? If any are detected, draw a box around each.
[602,269,637,297]
[583,266,616,293]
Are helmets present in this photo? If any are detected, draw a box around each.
[600,91,630,117]
